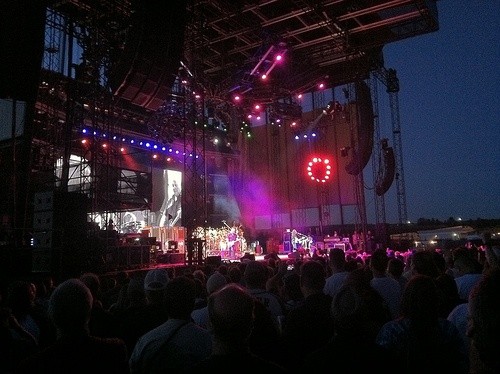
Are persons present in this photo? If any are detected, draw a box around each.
[0,220,500,374]
[367,253,401,320]
[159,179,182,228]
[324,248,350,297]
[366,230,374,241]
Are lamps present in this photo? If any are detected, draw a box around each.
[323,101,335,115]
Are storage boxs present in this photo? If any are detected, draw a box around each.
[334,244,346,252]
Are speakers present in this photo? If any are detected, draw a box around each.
[240,254,255,262]
[112,0,186,112]
[289,252,301,259]
[264,253,279,261]
[0,0,47,103]
[206,256,221,266]
[29,191,65,293]
[344,80,374,176]
[376,147,396,195]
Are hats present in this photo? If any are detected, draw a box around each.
[206,274,227,294]
[144,269,169,291]
